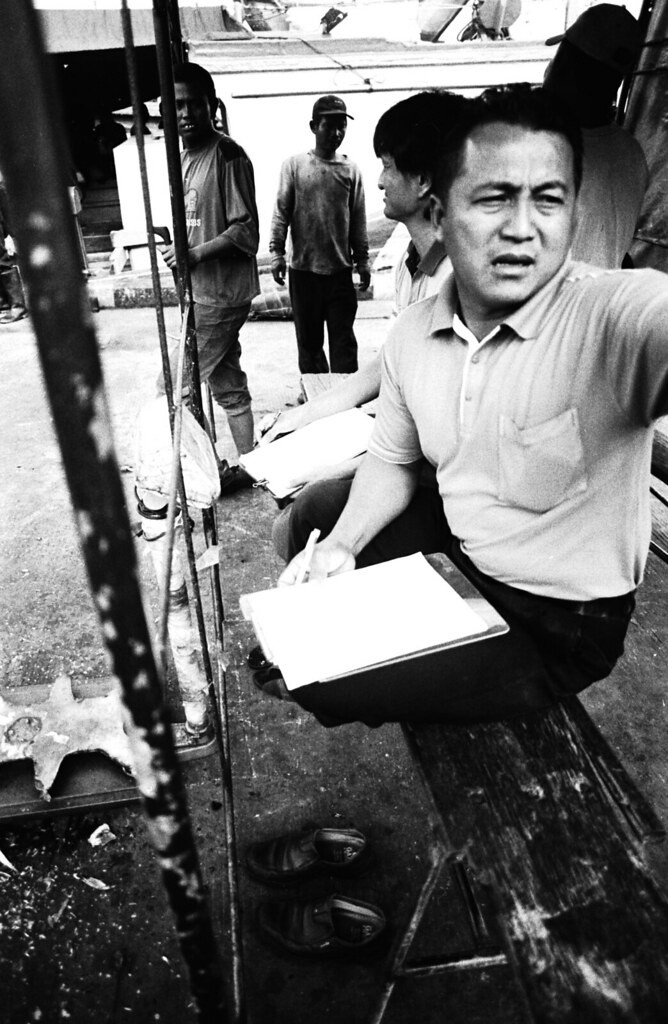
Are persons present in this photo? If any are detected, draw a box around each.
[277,81,668,729]
[541,3,649,265]
[241,92,468,684]
[268,96,370,370]
[159,62,267,450]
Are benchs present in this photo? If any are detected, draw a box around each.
[289,373,668,1024]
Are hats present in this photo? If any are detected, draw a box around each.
[313,95,354,121]
[545,4,643,68]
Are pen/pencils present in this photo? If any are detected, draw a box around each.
[295,527,322,587]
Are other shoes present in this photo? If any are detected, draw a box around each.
[217,459,254,496]
[251,667,283,689]
[246,645,273,670]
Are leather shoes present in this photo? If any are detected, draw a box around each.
[245,825,367,887]
[255,893,386,961]
